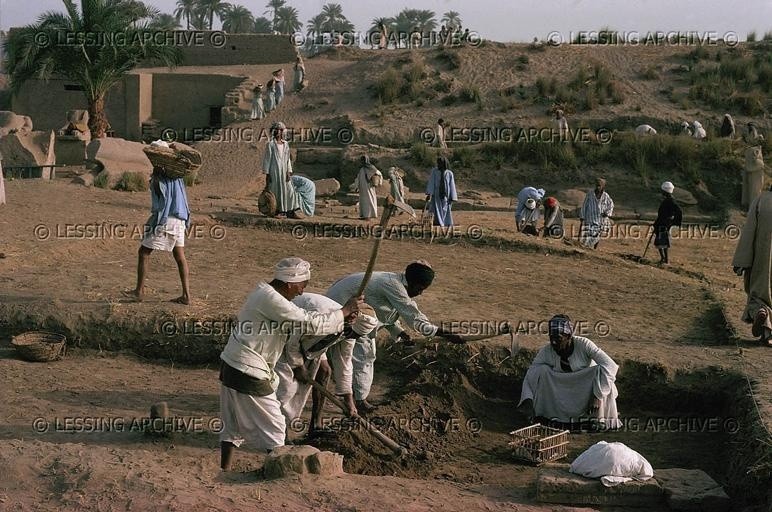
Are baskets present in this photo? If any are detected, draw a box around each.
[511,423,570,464]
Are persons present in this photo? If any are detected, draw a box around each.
[514,186,546,237]
[444,27,454,46]
[459,25,463,36]
[732,181,771,347]
[292,57,306,92]
[578,179,614,249]
[635,124,657,137]
[541,196,564,237]
[410,27,422,49]
[262,121,301,220]
[247,84,266,122]
[290,175,316,217]
[120,138,192,305]
[679,120,693,136]
[555,109,570,141]
[652,181,682,269]
[516,313,624,434]
[718,114,736,138]
[388,166,405,218]
[272,68,286,104]
[379,22,388,50]
[440,25,447,42]
[266,76,281,114]
[461,28,471,42]
[437,118,448,149]
[218,257,365,473]
[348,155,383,220]
[740,122,768,216]
[267,292,378,454]
[324,259,466,412]
[453,31,462,45]
[425,156,458,245]
[691,120,707,139]
